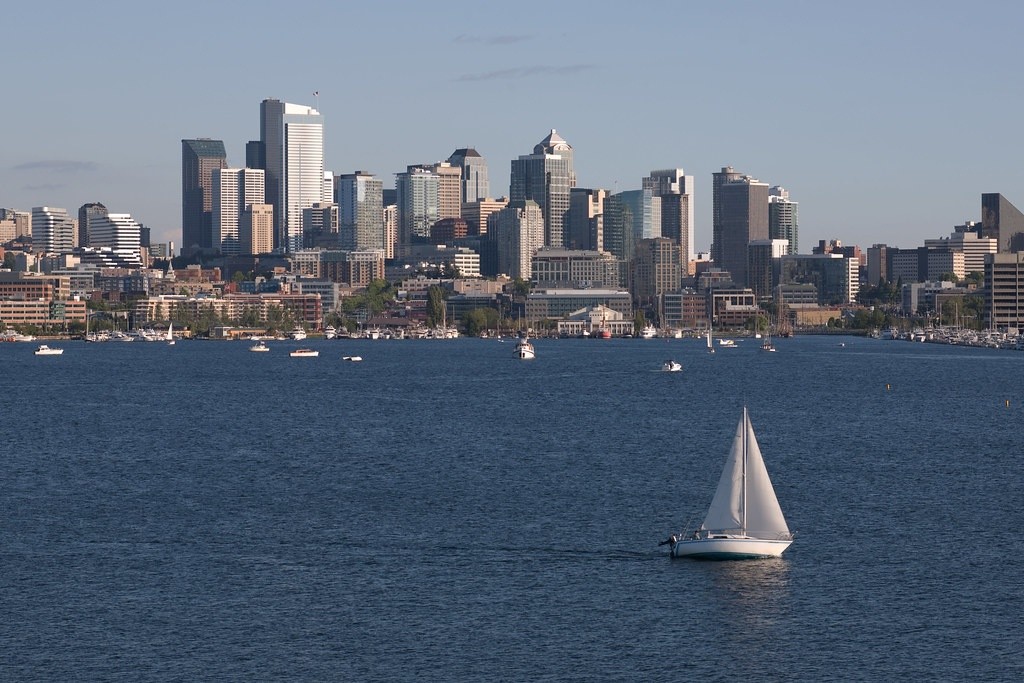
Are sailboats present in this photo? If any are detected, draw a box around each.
[165,320,175,345]
[661,405,794,559]
[707,331,715,354]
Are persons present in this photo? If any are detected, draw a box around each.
[690,530,701,540]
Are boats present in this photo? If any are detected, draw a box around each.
[325,323,459,340]
[621,334,632,338]
[289,347,319,357]
[577,325,591,339]
[250,344,270,352]
[598,318,611,339]
[111,331,134,342]
[638,323,655,340]
[659,360,683,372]
[33,345,63,354]
[342,355,363,361]
[719,339,739,348]
[290,325,307,339]
[1,330,33,342]
[870,302,1024,350]
[514,324,535,358]
[84,332,96,342]
[759,333,777,352]
